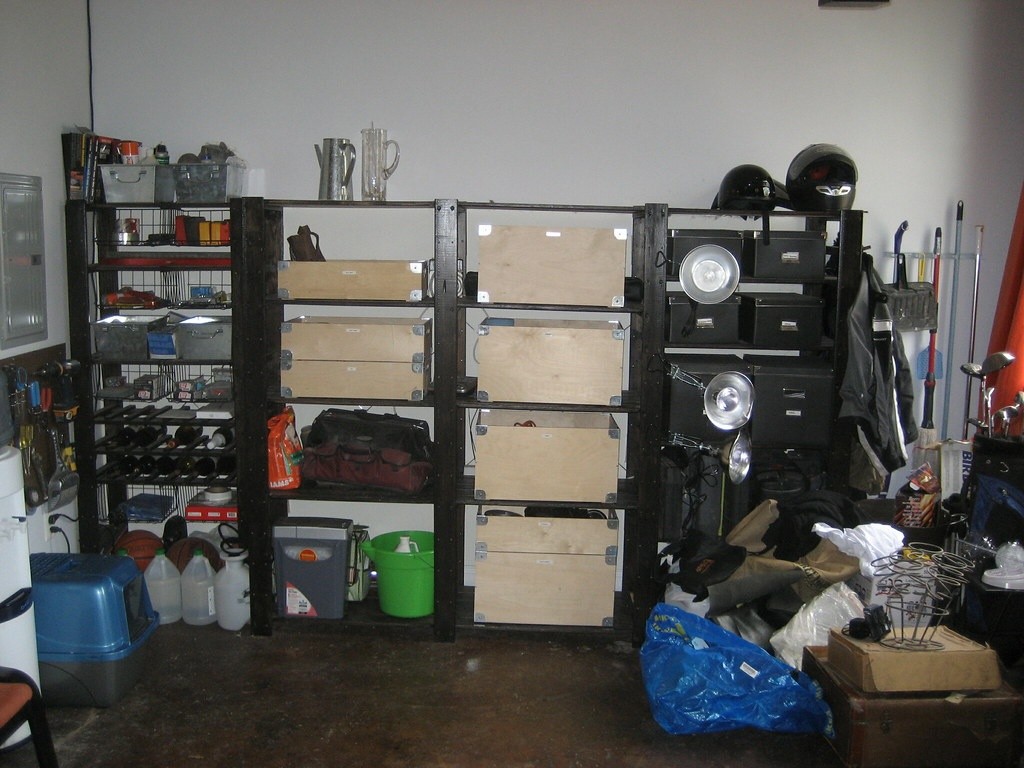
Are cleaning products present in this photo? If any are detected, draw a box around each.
[266,407,303,490]
[393,535,421,553]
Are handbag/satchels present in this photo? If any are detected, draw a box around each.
[301,408,433,494]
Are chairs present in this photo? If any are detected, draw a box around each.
[0,666,60,768]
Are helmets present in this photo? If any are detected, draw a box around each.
[718,164,776,212]
[786,144,858,210]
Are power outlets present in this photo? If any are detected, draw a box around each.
[42,511,56,543]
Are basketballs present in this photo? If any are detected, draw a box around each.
[113,530,163,571]
[168,537,223,572]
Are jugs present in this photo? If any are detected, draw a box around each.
[314,138,356,201]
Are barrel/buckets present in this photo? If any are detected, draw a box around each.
[357,530,434,618]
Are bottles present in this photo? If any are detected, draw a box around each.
[121,141,169,165]
[106,424,235,482]
[393,535,419,553]
[143,548,182,625]
[180,548,217,626]
[118,550,134,560]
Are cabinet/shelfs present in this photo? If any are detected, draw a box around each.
[65,195,251,556]
[243,197,456,641]
[666,208,868,530]
[435,199,667,646]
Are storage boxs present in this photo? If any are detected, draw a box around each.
[277,258,428,301]
[475,505,619,555]
[664,352,754,441]
[89,314,168,360]
[743,355,837,447]
[199,221,210,245]
[177,376,204,392]
[346,530,370,603]
[212,220,221,246]
[667,227,744,278]
[280,358,432,402]
[668,291,742,345]
[937,437,974,513]
[222,219,232,246]
[29,552,161,663]
[205,380,232,399]
[190,286,214,297]
[828,630,1005,693]
[154,164,247,205]
[176,316,232,360]
[803,646,1024,768]
[476,317,625,407]
[173,391,203,401]
[175,215,206,246]
[135,374,165,400]
[186,491,239,522]
[472,408,622,504]
[38,628,164,710]
[477,224,628,309]
[212,367,233,383]
[744,231,826,279]
[474,552,618,627]
[279,316,433,363]
[196,402,234,419]
[272,537,351,621]
[147,325,177,360]
[274,517,356,540]
[96,163,175,204]
[736,292,826,346]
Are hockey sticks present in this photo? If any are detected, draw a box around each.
[960,352,1024,440]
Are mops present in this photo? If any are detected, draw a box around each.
[911,225,941,482]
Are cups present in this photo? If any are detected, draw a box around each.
[361,128,400,202]
